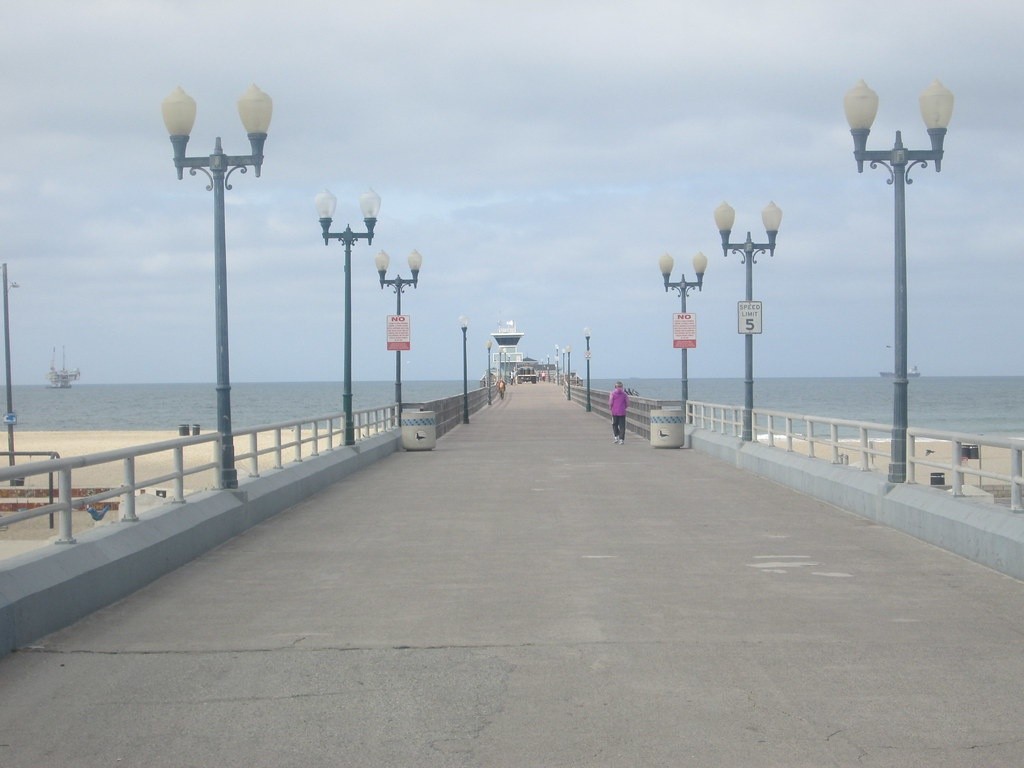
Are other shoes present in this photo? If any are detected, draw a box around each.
[619,439,624,444]
[613,436,618,443]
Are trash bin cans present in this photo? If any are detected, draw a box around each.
[962,445,979,459]
[930,473,945,485]
[179,424,190,436]
[193,424,201,436]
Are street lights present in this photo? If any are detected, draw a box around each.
[458,313,471,423]
[712,201,784,443]
[485,339,494,406]
[583,326,593,412]
[315,185,382,446]
[566,343,572,401]
[562,347,566,394]
[840,76,958,481]
[498,346,503,384]
[373,248,422,427]
[660,250,708,420]
[504,347,512,385]
[546,354,551,382]
[555,343,560,386]
[163,82,272,489]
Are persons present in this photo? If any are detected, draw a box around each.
[609,382,629,444]
[540,370,546,382]
[499,379,505,399]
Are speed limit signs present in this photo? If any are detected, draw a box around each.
[736,301,763,334]
